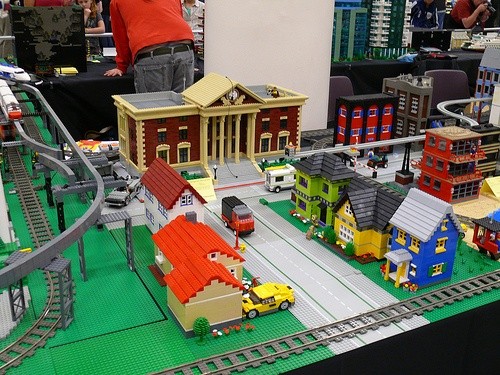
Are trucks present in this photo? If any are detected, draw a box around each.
[221,196,255,236]
[264,166,297,193]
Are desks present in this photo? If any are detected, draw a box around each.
[330,50,485,96]
[36,62,203,144]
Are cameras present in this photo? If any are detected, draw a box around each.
[486,2,497,13]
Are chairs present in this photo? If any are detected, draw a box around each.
[424,69,470,106]
[327,76,354,122]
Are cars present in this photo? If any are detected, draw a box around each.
[367,155,389,170]
[241,281,295,319]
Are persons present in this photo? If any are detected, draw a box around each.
[407,0,500,51]
[24,0,115,55]
[104,0,195,94]
[181,0,204,44]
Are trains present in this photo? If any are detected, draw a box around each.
[0,79,23,122]
[0,63,31,84]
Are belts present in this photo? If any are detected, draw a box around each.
[134,44,194,61]
